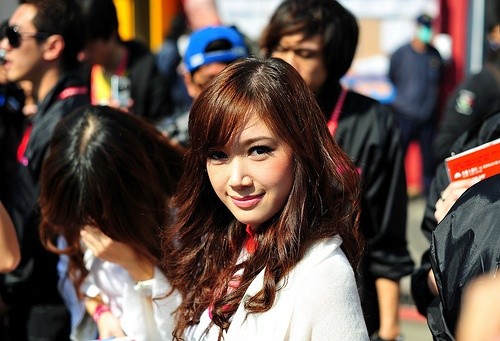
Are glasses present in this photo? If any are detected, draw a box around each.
[0,21,51,48]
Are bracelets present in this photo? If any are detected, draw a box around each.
[91,306,110,320]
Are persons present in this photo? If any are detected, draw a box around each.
[0,0,500,340]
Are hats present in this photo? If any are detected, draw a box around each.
[417,15,432,28]
[183,26,248,71]
